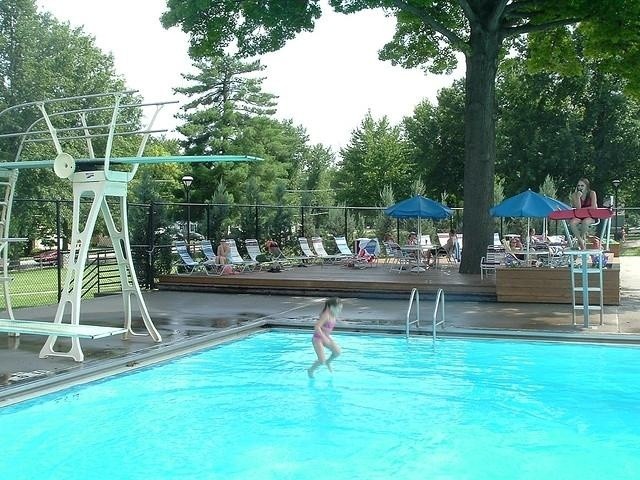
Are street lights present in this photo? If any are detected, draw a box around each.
[182,174,197,263]
[612,178,622,242]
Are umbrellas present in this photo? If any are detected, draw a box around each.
[488,188,574,266]
[382,194,455,270]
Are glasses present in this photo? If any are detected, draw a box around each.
[577,184,584,187]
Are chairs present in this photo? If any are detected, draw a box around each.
[169,232,604,277]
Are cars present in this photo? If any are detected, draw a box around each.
[31,251,75,268]
[153,222,206,244]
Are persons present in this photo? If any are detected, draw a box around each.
[510,236,525,261]
[568,178,599,252]
[405,230,422,262]
[306,298,342,381]
[215,238,233,275]
[620,226,626,241]
[529,228,551,248]
[425,228,456,267]
[385,232,409,260]
